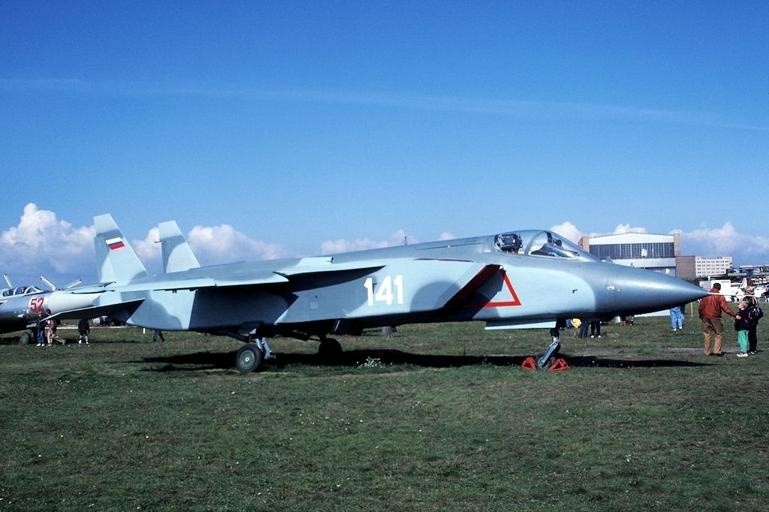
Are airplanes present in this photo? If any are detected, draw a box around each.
[38,208,759,372]
[0,272,98,346]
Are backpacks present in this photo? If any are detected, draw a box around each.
[56,319,61,326]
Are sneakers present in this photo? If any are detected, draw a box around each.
[47,343,52,346]
[736,350,757,358]
[41,344,45,346]
[590,335,602,338]
[36,344,41,346]
[673,326,683,331]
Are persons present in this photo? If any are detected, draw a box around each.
[77,319,91,345]
[152,329,164,341]
[34,311,46,347]
[670,306,684,332]
[734,300,752,358]
[739,286,760,356]
[699,282,743,355]
[570,318,589,338]
[589,318,602,338]
[42,309,66,346]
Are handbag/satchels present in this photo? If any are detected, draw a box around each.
[748,306,764,322]
